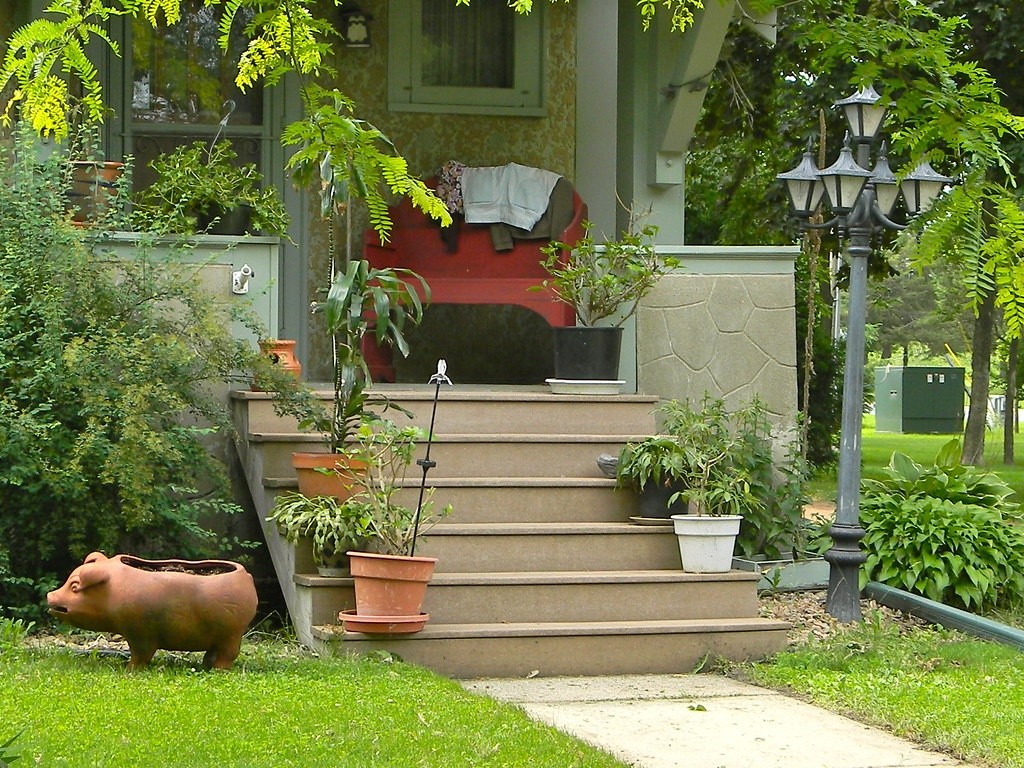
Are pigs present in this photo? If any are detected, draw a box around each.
[47,548,259,670]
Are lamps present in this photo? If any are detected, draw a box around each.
[334,1,374,49]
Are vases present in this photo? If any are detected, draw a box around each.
[251,337,302,391]
[46,553,262,667]
[57,158,125,231]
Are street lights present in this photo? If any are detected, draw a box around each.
[774,79,956,625]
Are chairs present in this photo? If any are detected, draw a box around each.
[356,161,589,384]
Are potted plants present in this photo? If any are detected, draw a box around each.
[613,429,695,526]
[291,257,431,509]
[266,417,453,637]
[650,393,769,576]
[523,184,688,399]
